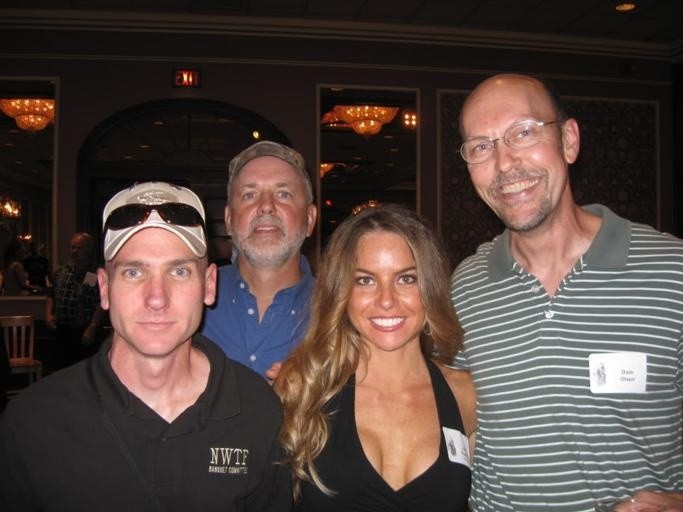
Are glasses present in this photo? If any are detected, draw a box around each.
[103,203,208,242]
[459,120,563,164]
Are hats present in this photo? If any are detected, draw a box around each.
[102,181,207,261]
[226,140,314,203]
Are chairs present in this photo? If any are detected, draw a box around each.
[0,314,42,402]
